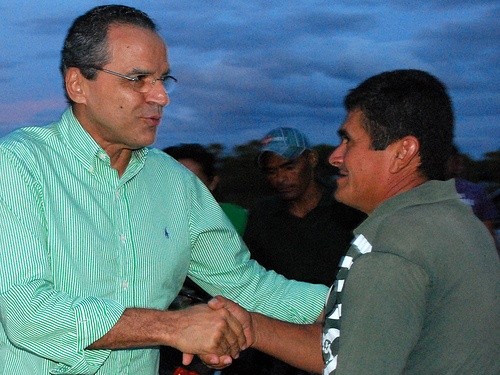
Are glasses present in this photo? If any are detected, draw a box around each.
[71,64,179,93]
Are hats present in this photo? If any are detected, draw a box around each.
[257,126,318,160]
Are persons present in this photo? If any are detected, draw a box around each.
[163,126,366,375]
[0,5,331,375]
[182,70,500,375]
[446,143,500,254]
